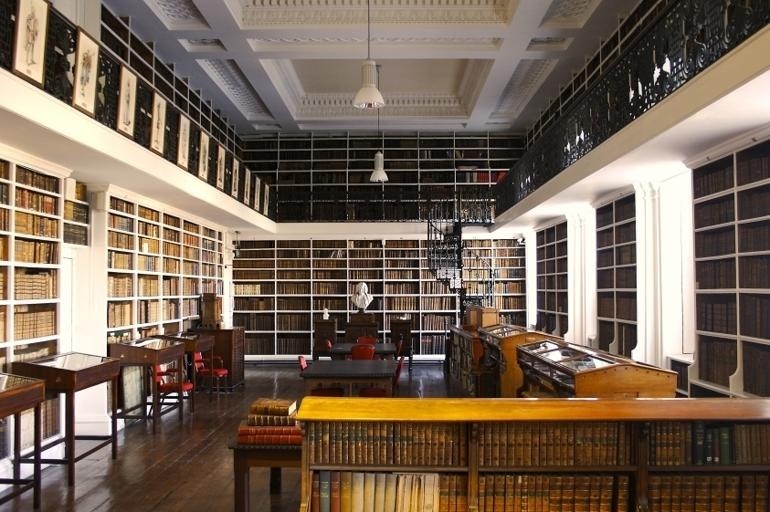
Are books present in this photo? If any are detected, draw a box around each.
[1,133,769,512]
